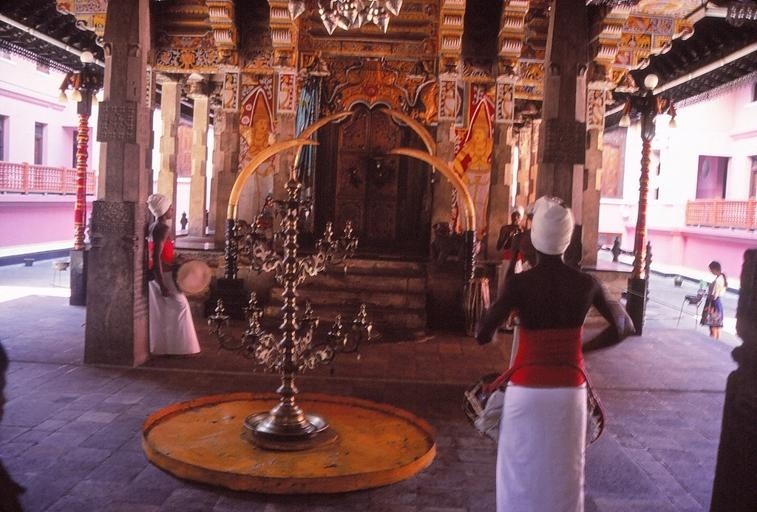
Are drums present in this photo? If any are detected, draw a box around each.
[171,252,213,296]
[461,371,507,439]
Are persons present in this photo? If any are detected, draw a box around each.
[223,74,235,110]
[498,83,512,119]
[440,81,454,117]
[147,193,202,360]
[701,260,728,340]
[277,76,292,110]
[0,342,26,511]
[505,200,537,326]
[235,91,279,225]
[473,194,638,512]
[495,204,525,333]
[449,102,494,242]
[585,88,604,128]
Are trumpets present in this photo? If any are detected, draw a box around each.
[514,221,521,239]
